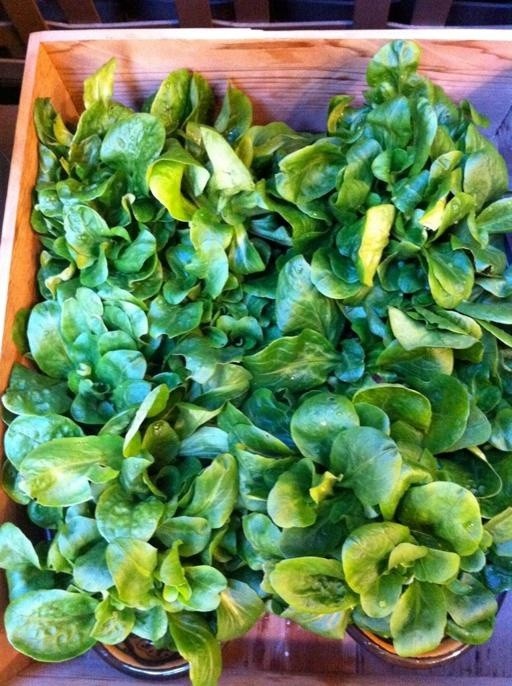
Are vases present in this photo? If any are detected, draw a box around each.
[96,643,192,680]
[348,623,471,670]
